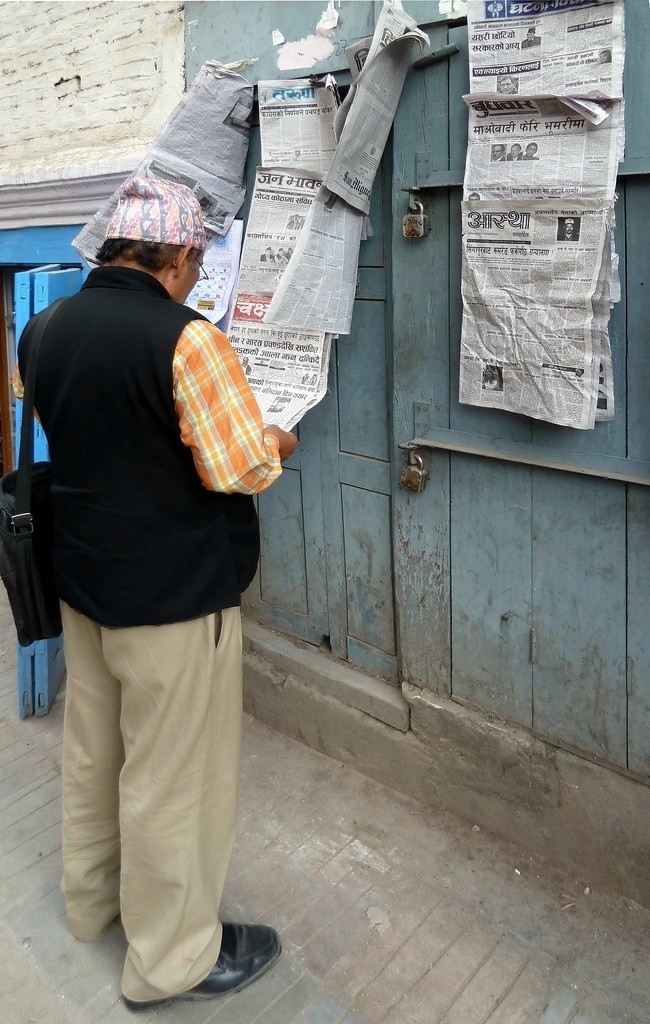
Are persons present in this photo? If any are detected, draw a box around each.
[0,177,298,1011]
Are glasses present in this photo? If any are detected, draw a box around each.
[187,251,208,282]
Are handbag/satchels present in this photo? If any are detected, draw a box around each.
[0,461,66,647]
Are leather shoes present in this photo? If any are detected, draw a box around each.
[126,923,282,1010]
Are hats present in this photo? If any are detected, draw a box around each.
[104,177,206,250]
